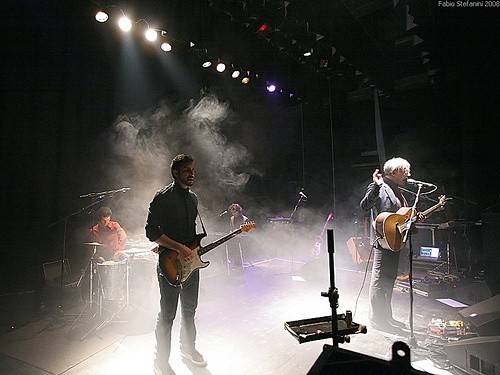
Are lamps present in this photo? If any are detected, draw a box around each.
[161,36,173,52]
[303,41,314,57]
[215,56,226,72]
[230,63,240,79]
[249,20,268,32]
[240,68,250,83]
[199,47,211,68]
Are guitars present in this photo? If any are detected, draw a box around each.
[312,212,335,256]
[158,221,256,287]
[374,194,448,252]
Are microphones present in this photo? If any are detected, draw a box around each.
[299,192,308,199]
[220,211,227,217]
[99,195,106,198]
[407,179,435,187]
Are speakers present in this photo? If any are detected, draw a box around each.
[447,293,500,375]
[42,258,70,283]
[346,237,374,265]
[299,258,329,285]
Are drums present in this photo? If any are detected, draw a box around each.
[95,255,158,307]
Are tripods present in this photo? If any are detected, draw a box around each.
[423,243,454,280]
[36,198,147,342]
[274,196,303,280]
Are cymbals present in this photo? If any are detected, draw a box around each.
[120,249,150,255]
[81,242,109,247]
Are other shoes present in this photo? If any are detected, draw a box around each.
[181,348,206,366]
[371,320,402,335]
[153,363,176,375]
[389,318,406,328]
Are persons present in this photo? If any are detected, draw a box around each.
[145,154,208,375]
[228,204,248,266]
[360,157,426,334]
[88,207,128,301]
